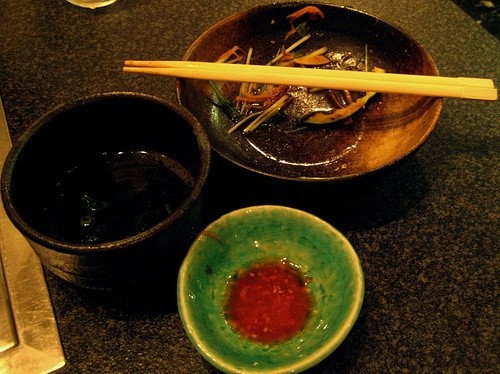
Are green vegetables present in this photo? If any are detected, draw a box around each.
[209,20,310,123]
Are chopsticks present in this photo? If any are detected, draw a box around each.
[122,57,498,103]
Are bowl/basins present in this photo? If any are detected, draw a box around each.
[0,91,213,292]
[177,204,368,374]
[176,0,444,186]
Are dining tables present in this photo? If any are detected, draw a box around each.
[0,0,500,374]
